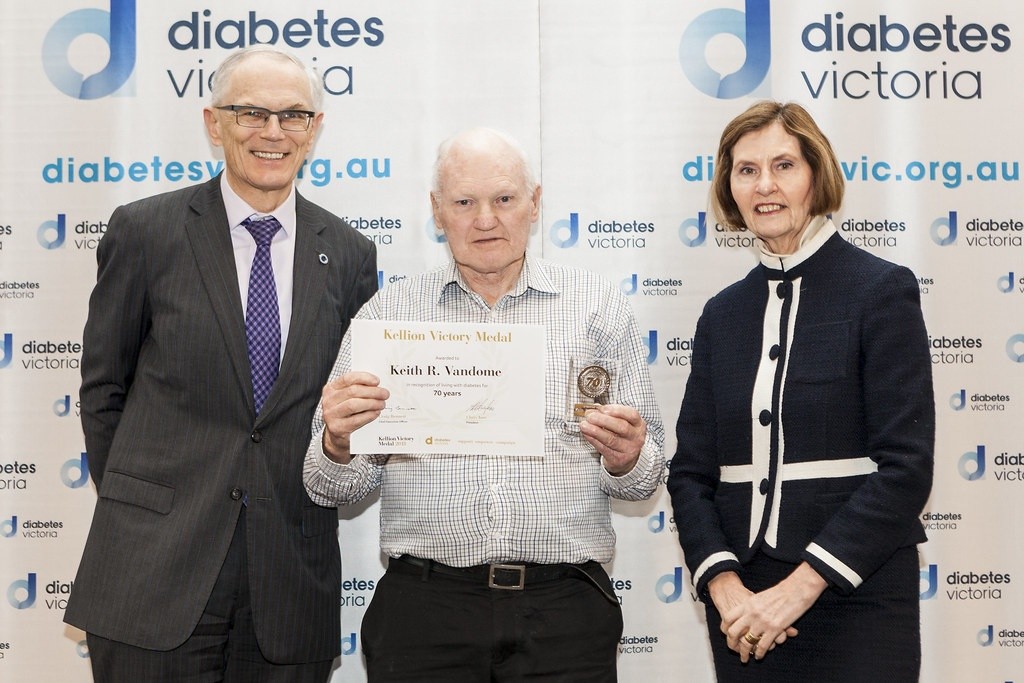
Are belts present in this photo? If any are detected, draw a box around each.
[397,552,618,605]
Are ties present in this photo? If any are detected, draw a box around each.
[238,216,283,421]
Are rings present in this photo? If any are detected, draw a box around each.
[744,633,760,644]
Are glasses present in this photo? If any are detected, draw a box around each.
[215,105,315,133]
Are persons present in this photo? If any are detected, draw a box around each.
[62,44,378,683]
[667,101,937,683]
[301,126,666,683]
